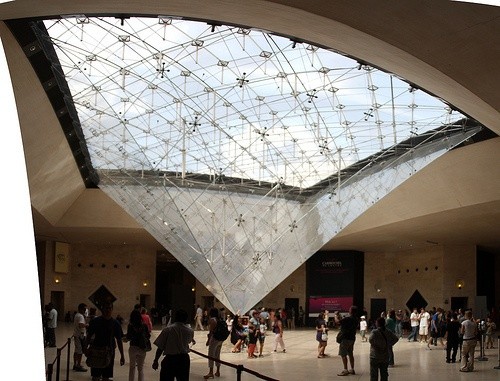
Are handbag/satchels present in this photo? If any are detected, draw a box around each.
[321,328,328,341]
[138,330,151,351]
[85,345,112,368]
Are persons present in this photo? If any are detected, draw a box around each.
[43,302,496,381]
[335,306,359,376]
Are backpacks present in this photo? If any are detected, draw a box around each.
[213,320,230,341]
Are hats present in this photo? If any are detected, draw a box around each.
[360,316,365,319]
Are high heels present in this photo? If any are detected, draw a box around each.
[204,373,220,379]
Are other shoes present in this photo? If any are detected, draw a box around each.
[232,349,240,353]
[73,365,88,372]
[271,350,286,354]
[259,355,263,357]
[337,369,356,376]
[318,354,328,358]
[48,344,56,347]
[361,337,367,342]
[248,355,257,359]
